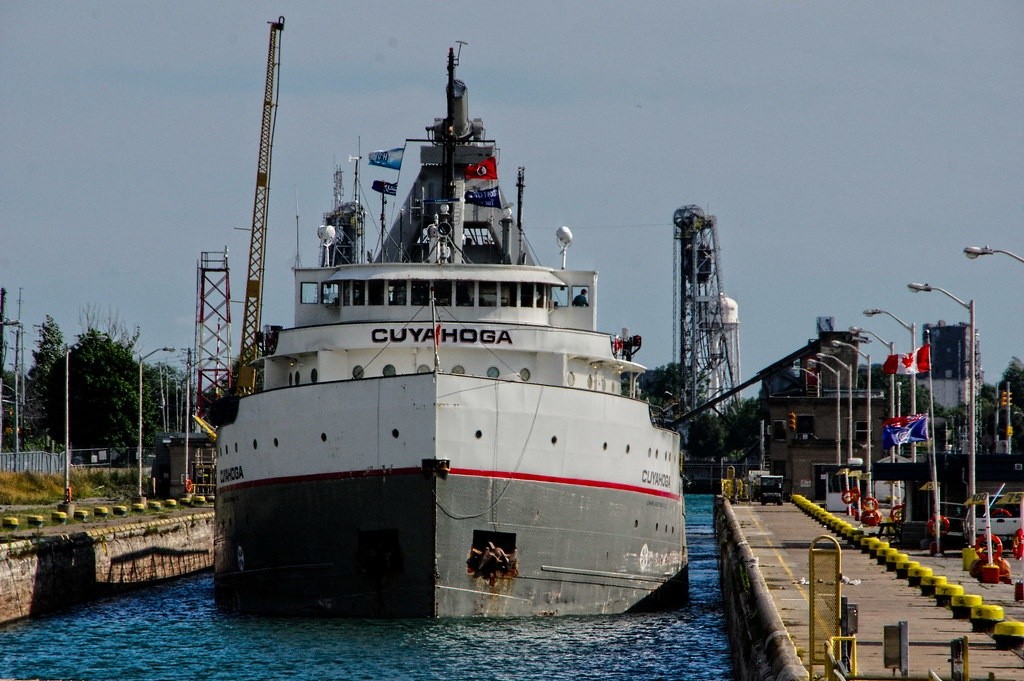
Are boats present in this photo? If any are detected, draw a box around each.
[933,490,1024,544]
[190,40,689,618]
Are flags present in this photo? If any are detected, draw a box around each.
[368,146,404,170]
[464,186,503,210]
[466,157,497,181]
[371,179,398,196]
[879,413,927,450]
[882,346,930,375]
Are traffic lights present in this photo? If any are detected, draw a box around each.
[1002,391,1007,406]
[787,414,796,432]
[1009,392,1013,405]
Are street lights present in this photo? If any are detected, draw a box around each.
[831,339,874,517]
[849,326,895,509]
[816,351,853,457]
[862,308,943,557]
[806,358,841,464]
[963,245,1024,262]
[907,283,977,548]
[137,346,176,497]
[64,335,108,502]
[788,367,820,397]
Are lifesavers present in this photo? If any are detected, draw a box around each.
[889,504,903,523]
[851,487,860,502]
[862,510,879,525]
[862,497,879,510]
[841,490,853,504]
[927,514,950,537]
[975,533,1004,562]
[991,508,1012,518]
[1012,527,1024,560]
[186,479,193,493]
[68,486,72,503]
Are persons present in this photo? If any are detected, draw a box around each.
[572,289,588,307]
